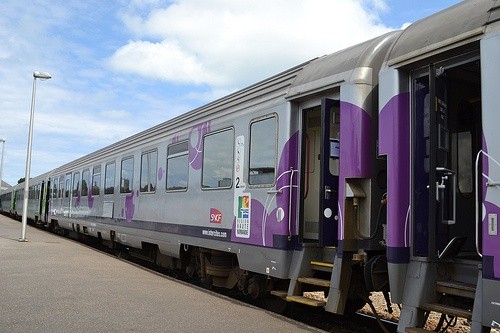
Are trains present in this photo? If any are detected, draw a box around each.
[0,0,500,331]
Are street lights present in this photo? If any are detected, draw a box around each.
[0,138,6,186]
[19,72,52,242]
